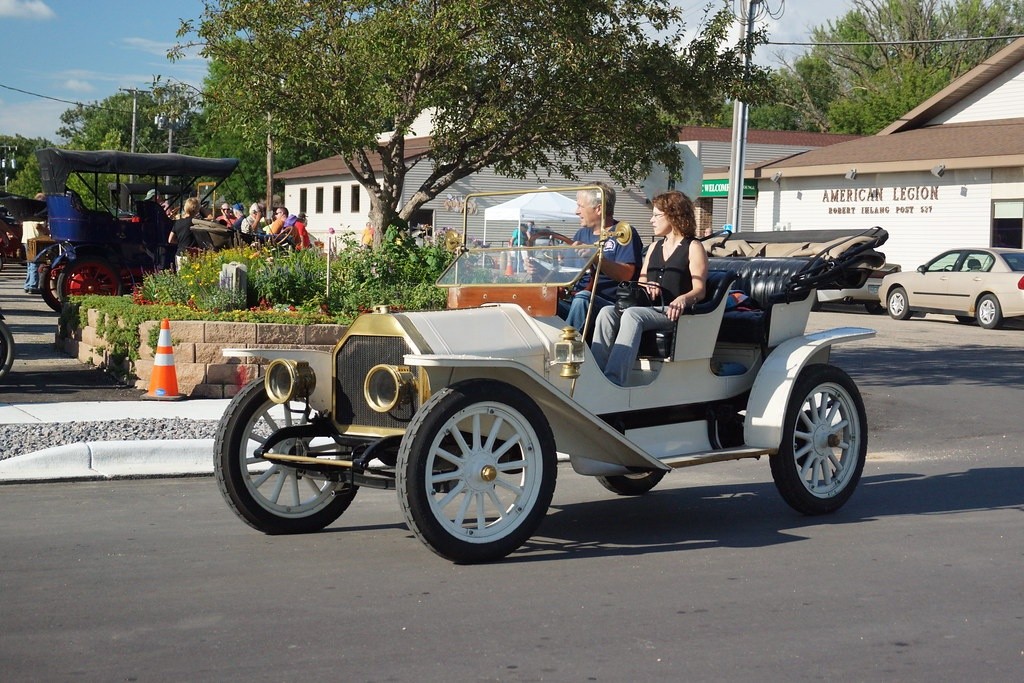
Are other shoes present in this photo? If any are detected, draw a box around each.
[25,288,41,294]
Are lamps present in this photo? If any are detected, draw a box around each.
[844,168,856,180]
[931,164,945,177]
[769,170,782,182]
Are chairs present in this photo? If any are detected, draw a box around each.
[966,259,984,272]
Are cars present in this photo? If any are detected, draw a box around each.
[208,187,872,566]
[809,259,901,313]
[38,149,295,311]
[880,243,1024,331]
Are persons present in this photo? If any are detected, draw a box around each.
[361,222,373,249]
[141,189,314,277]
[591,189,710,386]
[524,183,644,336]
[20,192,47,294]
[508,220,535,273]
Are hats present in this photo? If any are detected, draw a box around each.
[232,203,244,210]
[298,212,308,217]
[144,189,159,200]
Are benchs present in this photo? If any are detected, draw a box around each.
[707,257,826,347]
[637,268,737,359]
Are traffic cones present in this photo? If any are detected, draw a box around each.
[140,319,184,405]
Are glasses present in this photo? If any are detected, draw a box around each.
[221,208,231,211]
[275,212,284,215]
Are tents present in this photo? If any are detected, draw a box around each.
[482,185,580,269]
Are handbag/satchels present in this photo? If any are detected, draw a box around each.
[614,281,664,318]
